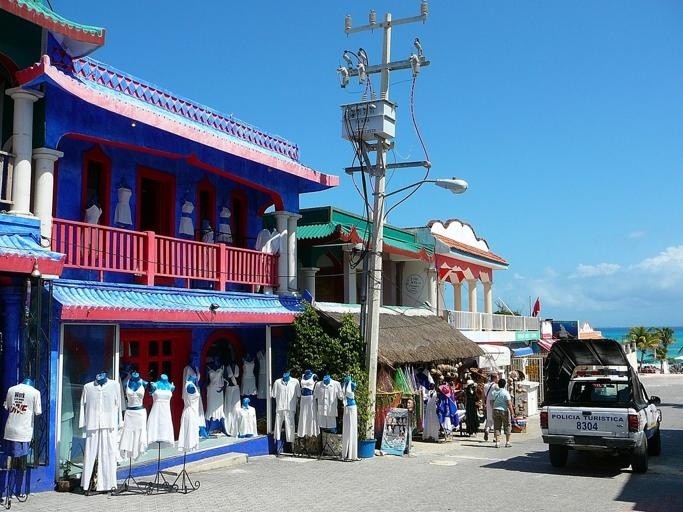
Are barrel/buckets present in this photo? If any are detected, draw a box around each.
[357,438,377,458]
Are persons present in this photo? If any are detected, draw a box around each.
[490,379,517,449]
[179,190,197,237]
[255,219,280,292]
[0,376,43,460]
[118,369,151,465]
[114,177,134,226]
[77,195,103,260]
[218,204,234,245]
[406,398,416,411]
[78,368,124,492]
[271,371,302,444]
[179,375,200,451]
[463,379,480,435]
[483,373,502,442]
[143,371,177,448]
[202,219,215,244]
[231,396,259,440]
[341,376,363,462]
[255,346,268,401]
[298,369,322,440]
[241,352,258,398]
[311,374,343,432]
[180,350,210,442]
[203,355,229,431]
[223,356,242,439]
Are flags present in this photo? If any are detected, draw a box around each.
[532,298,541,317]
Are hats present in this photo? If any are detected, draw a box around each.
[439,385,452,397]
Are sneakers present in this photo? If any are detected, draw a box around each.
[484,430,512,447]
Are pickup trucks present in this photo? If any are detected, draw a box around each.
[538,367,664,474]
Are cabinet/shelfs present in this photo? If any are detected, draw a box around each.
[515,381,539,417]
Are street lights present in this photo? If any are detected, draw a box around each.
[355,176,472,452]
